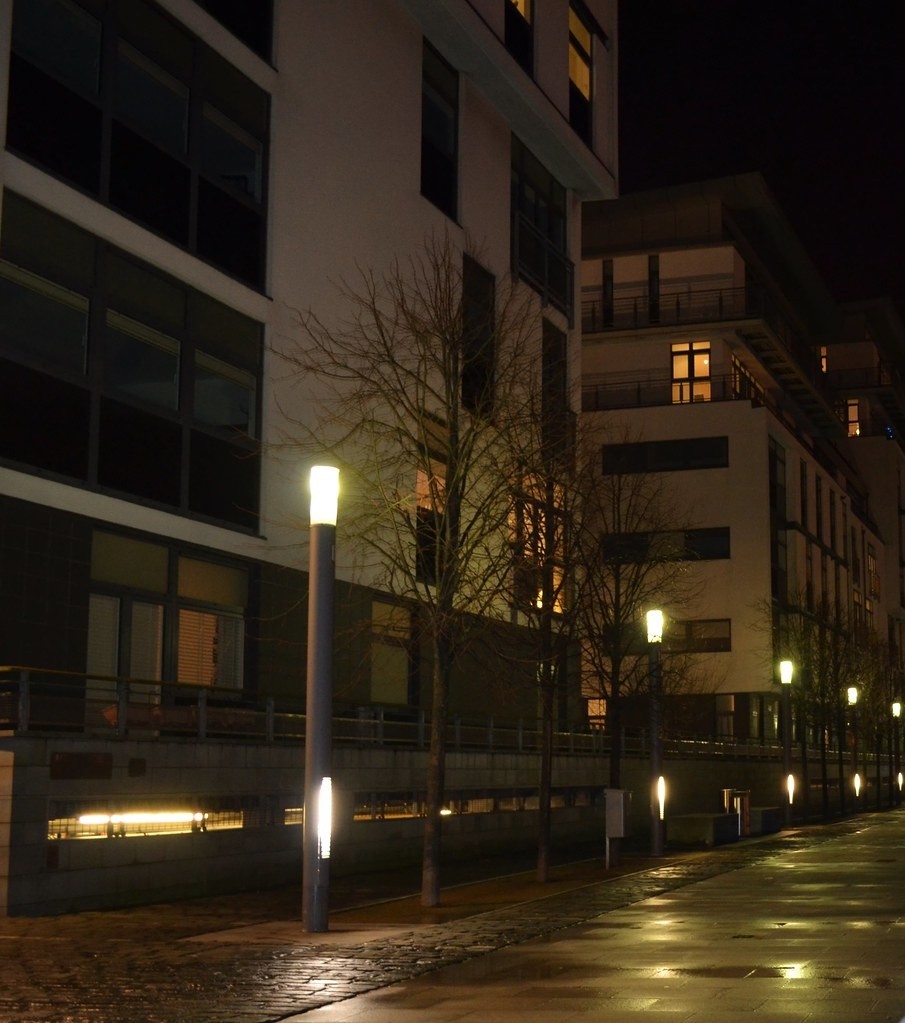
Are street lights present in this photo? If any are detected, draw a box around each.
[299,465,343,933]
[646,608,667,855]
[778,657,798,823]
[844,681,864,816]
[888,698,903,804]
